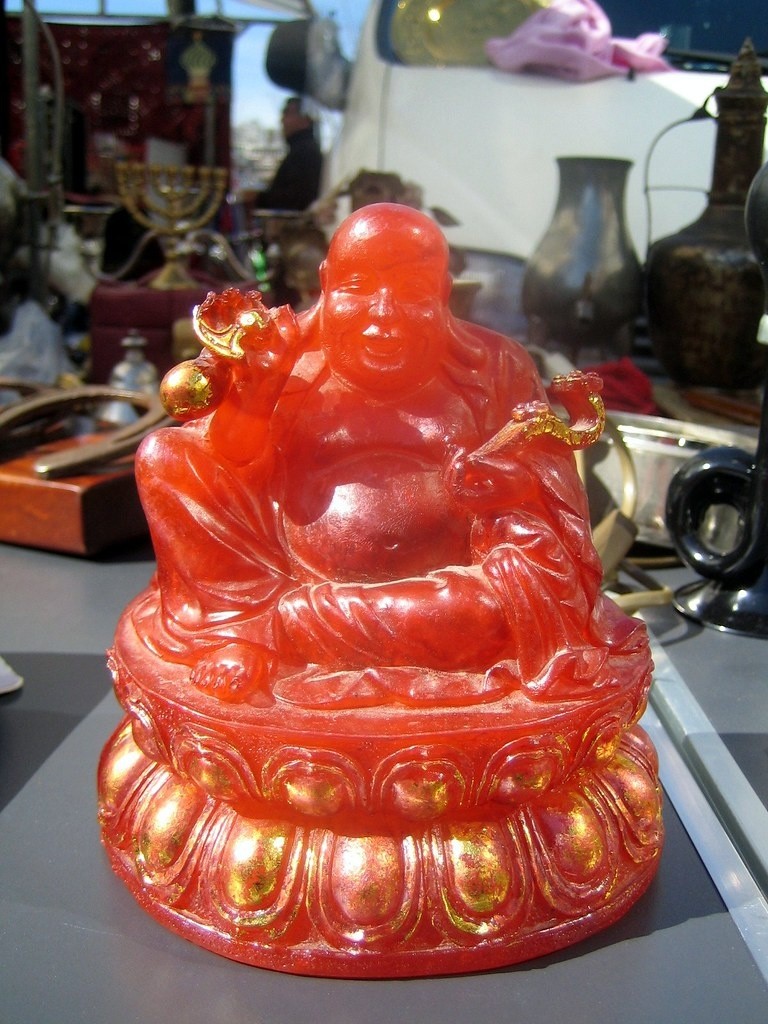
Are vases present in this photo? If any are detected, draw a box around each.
[519,156,645,378]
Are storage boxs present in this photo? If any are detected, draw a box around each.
[92,282,273,375]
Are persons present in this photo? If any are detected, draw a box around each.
[136,201,601,666]
[256,98,322,211]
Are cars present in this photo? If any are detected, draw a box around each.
[1,0,768,302]
